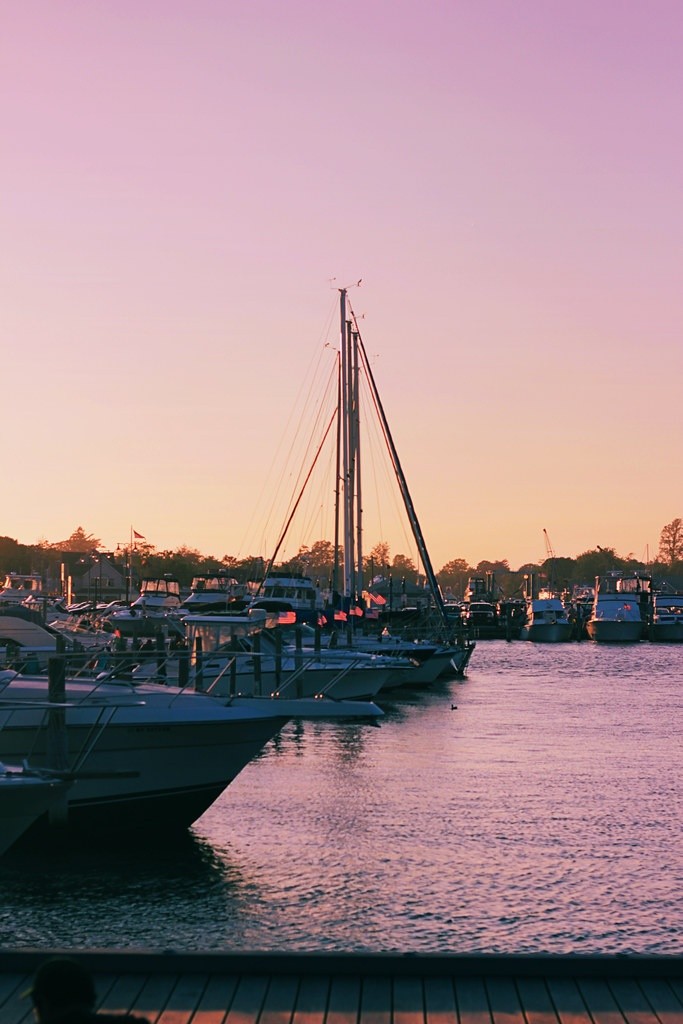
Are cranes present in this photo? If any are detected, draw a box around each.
[543,528,567,599]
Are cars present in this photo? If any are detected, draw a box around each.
[442,604,460,623]
[67,601,109,615]
[422,606,441,623]
[467,603,496,625]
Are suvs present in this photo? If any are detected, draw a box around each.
[497,603,523,626]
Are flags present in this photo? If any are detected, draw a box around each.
[349,605,363,616]
[334,609,347,621]
[623,603,631,610]
[365,608,379,618]
[368,591,386,604]
[133,530,145,538]
[317,612,328,627]
[277,612,296,624]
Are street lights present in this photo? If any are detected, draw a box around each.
[115,542,139,605]
[92,549,111,601]
[80,552,96,600]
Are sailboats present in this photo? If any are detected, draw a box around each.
[181,286,479,700]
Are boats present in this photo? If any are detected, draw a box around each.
[139,573,248,628]
[0,655,385,882]
[584,569,646,645]
[165,571,323,633]
[100,576,188,633]
[463,577,491,602]
[0,574,178,669]
[643,592,683,639]
[518,598,569,642]
